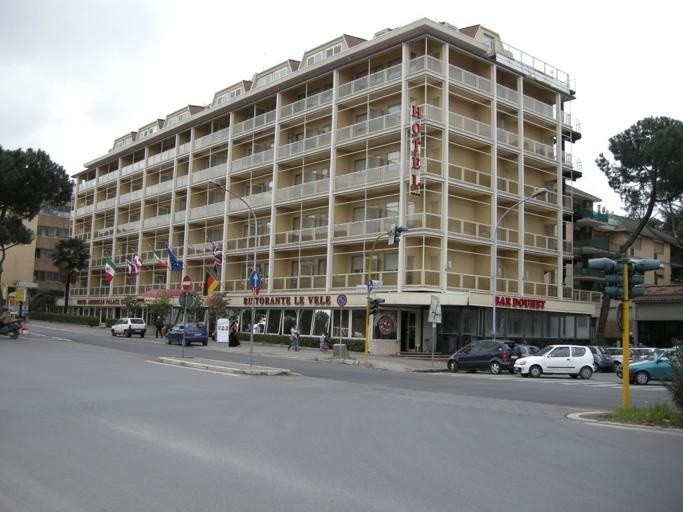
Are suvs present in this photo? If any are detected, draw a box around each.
[110,316,148,339]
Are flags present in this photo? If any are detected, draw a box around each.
[213,244,222,265]
[132,251,143,275]
[153,251,167,269]
[202,273,219,295]
[127,263,135,276]
[165,242,182,271]
[104,254,116,282]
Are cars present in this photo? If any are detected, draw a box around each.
[513,344,597,379]
[614,348,680,385]
[332,326,363,337]
[164,322,208,347]
[513,341,680,372]
[444,338,519,378]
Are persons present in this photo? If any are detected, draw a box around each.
[155,315,164,338]
[229,321,240,347]
[0,305,12,328]
[319,329,326,350]
[287,323,299,351]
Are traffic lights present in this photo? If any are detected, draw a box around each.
[627,256,661,300]
[368,299,378,315]
[586,256,624,302]
[393,225,408,243]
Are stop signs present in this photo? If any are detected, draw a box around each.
[181,274,192,292]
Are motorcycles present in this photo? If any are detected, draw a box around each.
[0,318,24,341]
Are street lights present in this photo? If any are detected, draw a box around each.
[207,178,259,356]
[491,186,550,341]
